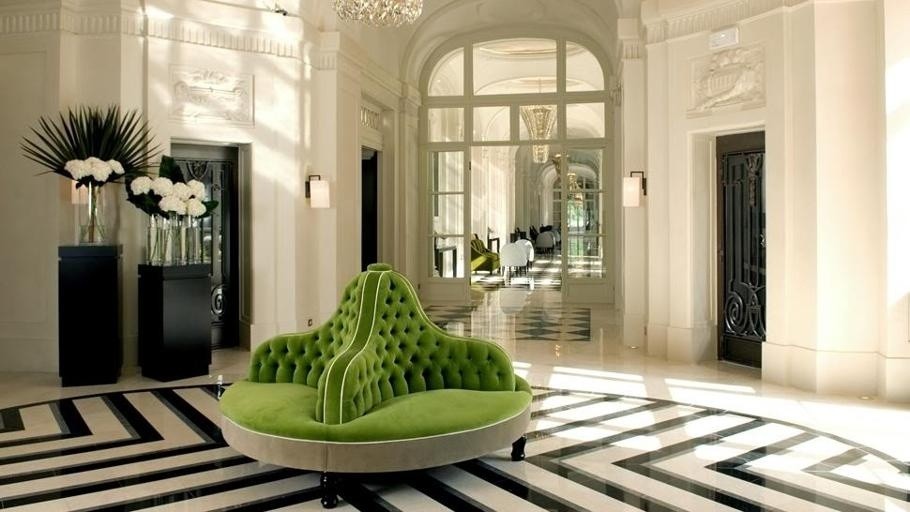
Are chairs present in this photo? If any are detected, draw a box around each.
[471,239,499,276]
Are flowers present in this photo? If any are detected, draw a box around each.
[18,99,164,240]
[124,155,219,263]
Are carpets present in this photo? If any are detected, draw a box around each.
[0,381,910,512]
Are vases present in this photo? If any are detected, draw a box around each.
[79,187,112,246]
[146,214,202,268]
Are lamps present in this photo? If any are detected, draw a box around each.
[622,170,647,208]
[519,80,557,164]
[306,174,330,209]
[331,0,425,30]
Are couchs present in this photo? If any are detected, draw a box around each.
[217,259,540,510]
